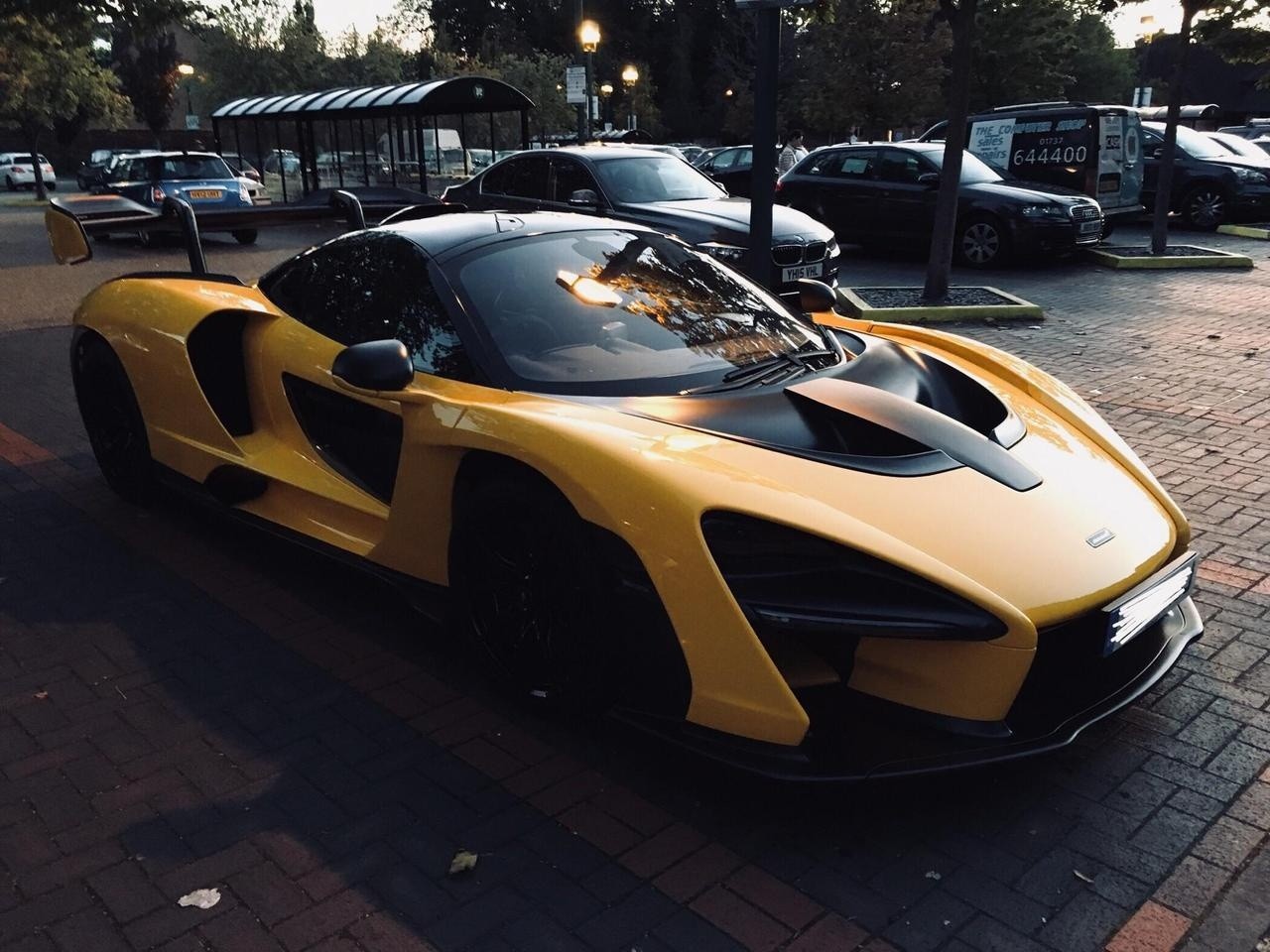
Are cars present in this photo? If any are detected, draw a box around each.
[1139,120,1270,233]
[74,125,526,251]
[774,140,1105,272]
[585,136,809,200]
[436,143,846,305]
[0,150,56,193]
[1196,116,1270,159]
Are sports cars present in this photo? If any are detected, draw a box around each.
[42,181,1206,793]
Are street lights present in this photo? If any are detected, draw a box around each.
[1136,13,1156,109]
[577,16,601,145]
[179,61,197,151]
[600,79,615,131]
[621,63,640,129]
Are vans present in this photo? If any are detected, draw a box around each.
[913,99,1147,240]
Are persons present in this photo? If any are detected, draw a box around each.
[779,129,805,176]
